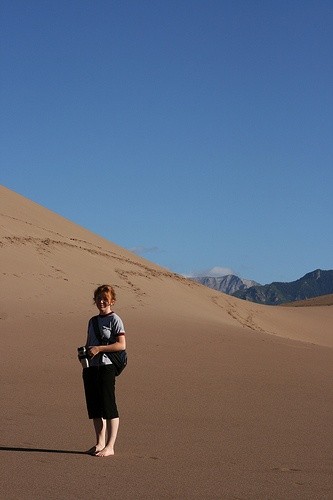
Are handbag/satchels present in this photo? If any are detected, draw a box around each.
[91,315,128,377]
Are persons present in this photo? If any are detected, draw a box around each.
[78,285,126,457]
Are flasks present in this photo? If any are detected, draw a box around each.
[77,346,90,368]
